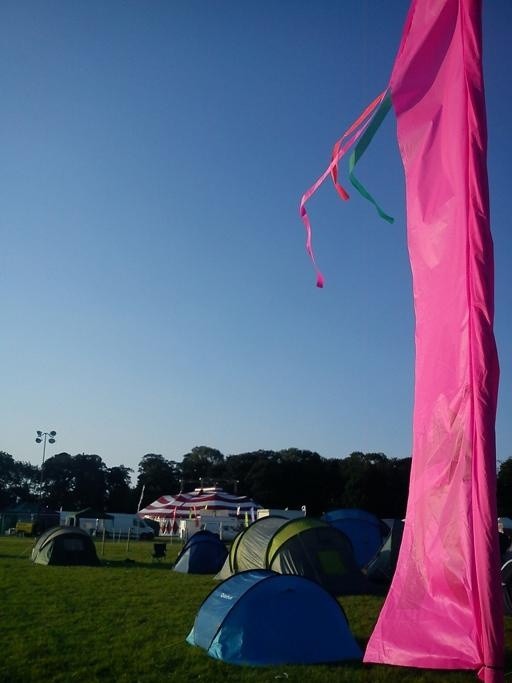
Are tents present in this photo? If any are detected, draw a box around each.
[31,525,100,565]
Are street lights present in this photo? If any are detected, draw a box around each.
[35,430,56,500]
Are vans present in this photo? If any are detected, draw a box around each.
[179,516,243,546]
[105,513,155,541]
